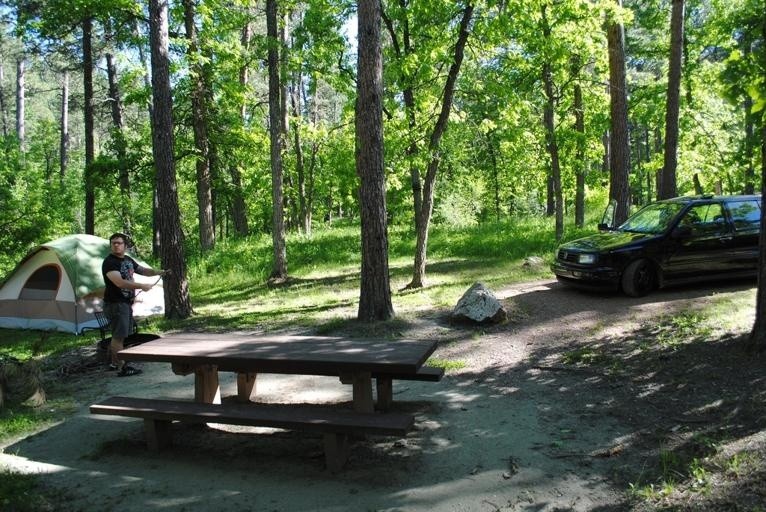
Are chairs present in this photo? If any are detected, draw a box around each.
[92,309,139,339]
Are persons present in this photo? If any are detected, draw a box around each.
[103,234,167,379]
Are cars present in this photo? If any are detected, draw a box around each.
[550,194,762,298]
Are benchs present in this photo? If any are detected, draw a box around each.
[171,361,445,408]
[90,396,415,477]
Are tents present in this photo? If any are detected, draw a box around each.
[1,234,166,336]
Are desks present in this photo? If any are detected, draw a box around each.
[117,331,438,414]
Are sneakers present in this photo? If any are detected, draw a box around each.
[108,363,119,371]
[116,365,143,377]
